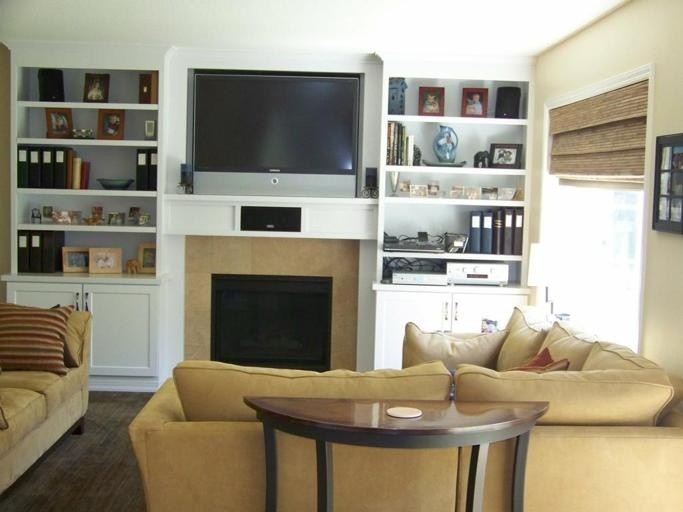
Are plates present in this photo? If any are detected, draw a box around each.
[423,160,467,167]
[387,407,422,418]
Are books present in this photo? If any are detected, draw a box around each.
[469,208,523,255]
[386,121,414,166]
[18,230,65,274]
[17,143,91,190]
[137,149,157,191]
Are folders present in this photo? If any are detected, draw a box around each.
[53,147,73,188]
[41,147,53,188]
[503,208,514,254]
[514,210,523,254]
[42,231,65,272]
[29,231,42,273]
[149,149,157,191]
[136,148,149,191]
[18,230,29,272]
[17,146,28,188]
[28,147,41,188]
[482,212,494,253]
[469,211,481,253]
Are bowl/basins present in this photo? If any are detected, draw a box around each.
[97,178,135,190]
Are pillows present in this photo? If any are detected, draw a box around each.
[509,347,569,373]
[403,320,509,370]
[0,303,74,376]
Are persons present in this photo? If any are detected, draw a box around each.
[87,83,103,99]
[466,99,477,115]
[424,94,439,112]
[56,117,68,130]
[105,118,120,133]
[496,148,512,164]
[472,94,482,116]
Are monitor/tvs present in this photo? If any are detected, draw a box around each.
[187,69,366,198]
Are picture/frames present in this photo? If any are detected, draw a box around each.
[87,247,122,275]
[487,143,523,169]
[82,72,109,103]
[96,108,124,140]
[137,243,155,274]
[460,87,488,118]
[45,108,71,139]
[61,246,88,273]
[417,87,446,117]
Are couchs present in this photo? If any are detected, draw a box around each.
[127,304,683,511]
[0,301,92,500]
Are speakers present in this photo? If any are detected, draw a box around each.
[365,166,379,191]
[181,163,187,186]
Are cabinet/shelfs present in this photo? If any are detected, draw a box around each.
[374,50,532,369]
[7,46,163,393]
